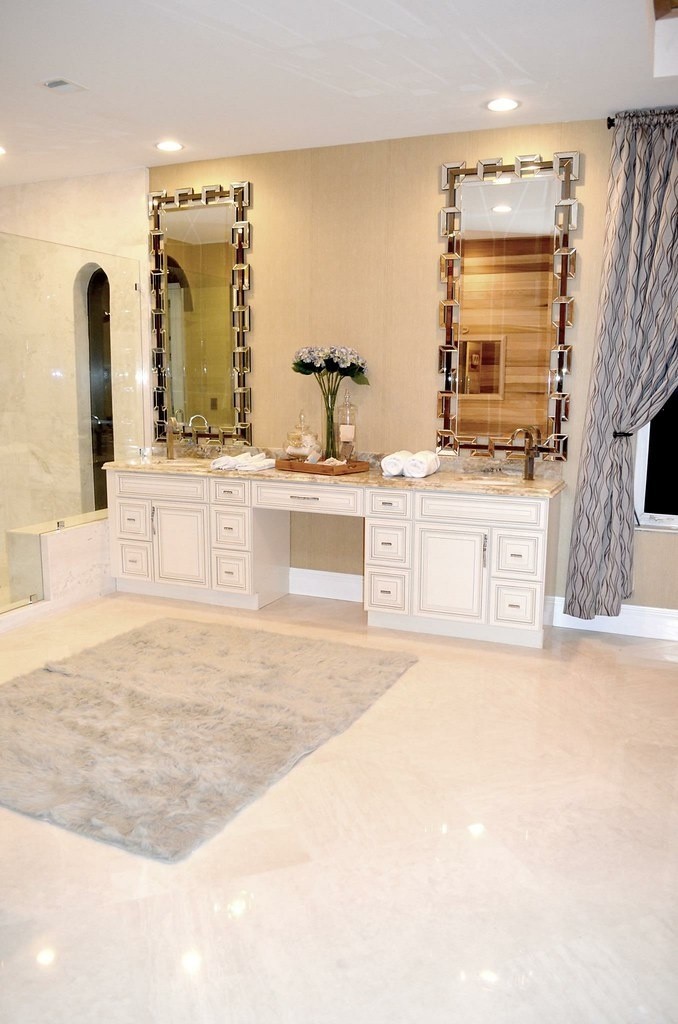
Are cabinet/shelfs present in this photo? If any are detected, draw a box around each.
[105,471,561,650]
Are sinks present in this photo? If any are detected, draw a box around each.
[447,477,524,485]
[152,460,207,467]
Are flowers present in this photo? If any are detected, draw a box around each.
[291,347,366,396]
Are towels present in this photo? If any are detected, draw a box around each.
[210,453,276,471]
[379,449,440,477]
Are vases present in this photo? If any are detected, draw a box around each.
[320,397,340,459]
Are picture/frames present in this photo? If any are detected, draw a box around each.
[460,335,507,403]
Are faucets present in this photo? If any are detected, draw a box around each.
[506,428,540,480]
[530,424,551,447]
[174,408,186,423]
[166,416,182,459]
[189,414,207,427]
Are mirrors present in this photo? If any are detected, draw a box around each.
[435,149,581,460]
[147,179,252,446]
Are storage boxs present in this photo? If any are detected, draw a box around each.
[275,458,370,476]
